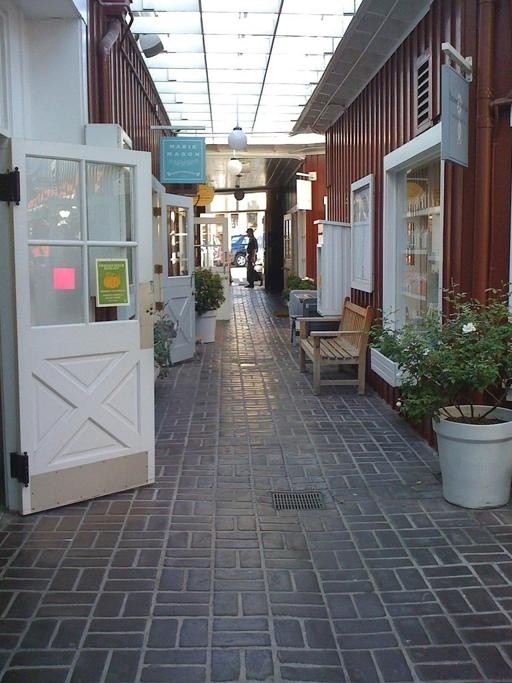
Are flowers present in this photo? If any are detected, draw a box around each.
[378,280,512,419]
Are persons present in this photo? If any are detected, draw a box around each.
[244,227,264,288]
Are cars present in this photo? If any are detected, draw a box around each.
[231,234,249,267]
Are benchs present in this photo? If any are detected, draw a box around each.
[297,296,373,396]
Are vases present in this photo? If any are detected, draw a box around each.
[431,404,512,510]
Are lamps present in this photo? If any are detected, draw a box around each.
[227,99,247,175]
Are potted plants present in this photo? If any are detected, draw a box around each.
[194,268,226,343]
